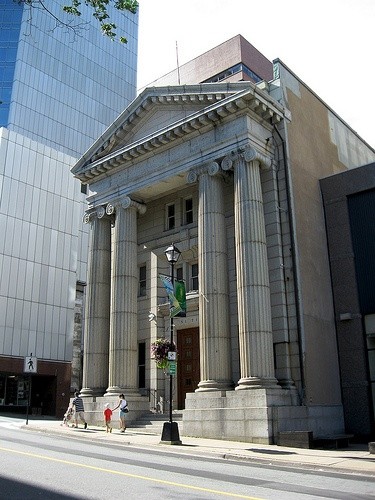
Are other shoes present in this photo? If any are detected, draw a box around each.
[84,423,87,429]
[120,427,126,433]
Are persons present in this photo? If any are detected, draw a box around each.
[71,392,87,429]
[63,407,72,424]
[35,393,42,407]
[111,393,127,432]
[104,403,112,432]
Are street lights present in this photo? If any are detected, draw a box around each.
[157,243,182,446]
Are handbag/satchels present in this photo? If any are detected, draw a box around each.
[122,408,129,413]
